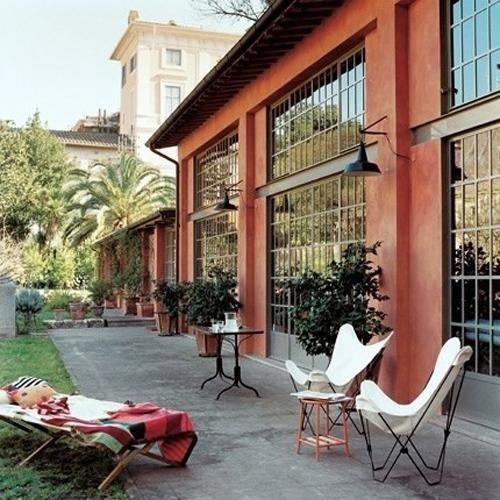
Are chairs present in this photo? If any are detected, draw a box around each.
[283,323,473,487]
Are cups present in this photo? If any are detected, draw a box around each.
[210,321,224,332]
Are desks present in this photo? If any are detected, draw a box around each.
[194,323,265,400]
[1,389,189,493]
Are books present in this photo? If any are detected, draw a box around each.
[289,390,354,403]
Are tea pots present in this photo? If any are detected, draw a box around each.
[223,313,242,332]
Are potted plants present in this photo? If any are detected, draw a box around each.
[275,238,396,412]
[49,230,245,358]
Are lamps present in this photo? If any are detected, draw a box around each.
[212,177,245,211]
[343,116,389,176]
